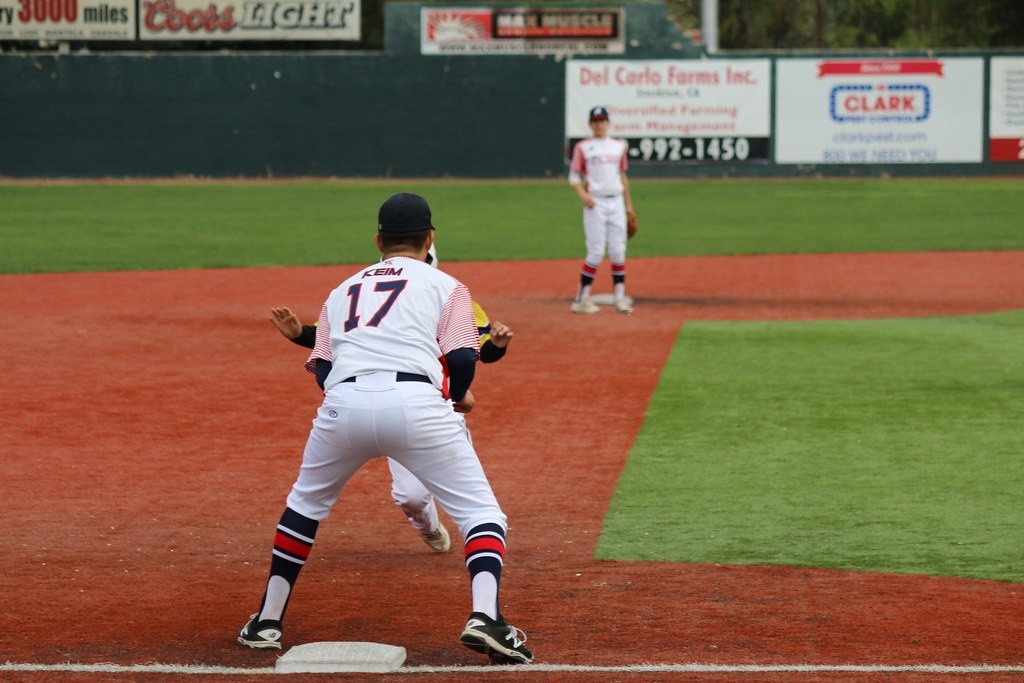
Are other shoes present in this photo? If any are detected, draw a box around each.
[570,299,601,313]
[615,301,633,314]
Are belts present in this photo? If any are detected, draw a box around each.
[341,372,432,384]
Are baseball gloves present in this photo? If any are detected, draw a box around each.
[627,211,637,240]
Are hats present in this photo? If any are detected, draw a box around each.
[378,192,436,232]
[590,107,608,120]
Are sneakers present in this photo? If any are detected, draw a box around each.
[238,613,283,649]
[460,611,533,663]
[419,522,450,553]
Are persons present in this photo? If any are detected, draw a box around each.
[269,239,513,555]
[237,192,536,665]
[568,106,635,314]
